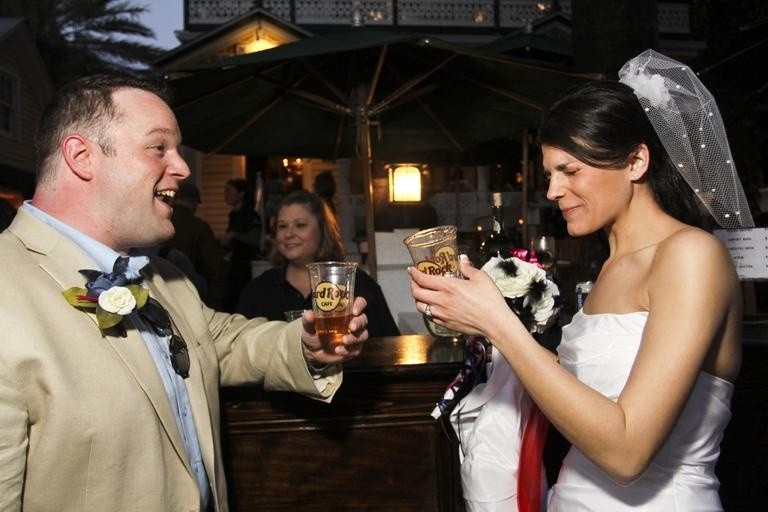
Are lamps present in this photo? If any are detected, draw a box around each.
[385,161,424,206]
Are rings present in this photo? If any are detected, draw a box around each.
[424,305,431,318]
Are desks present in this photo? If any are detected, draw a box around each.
[215,329,476,511]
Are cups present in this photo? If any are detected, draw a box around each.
[403,223,467,339]
[305,260,358,334]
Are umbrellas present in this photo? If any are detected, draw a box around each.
[148,9,614,280]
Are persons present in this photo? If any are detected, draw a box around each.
[157,182,220,311]
[408,51,756,511]
[240,189,400,339]
[220,179,263,315]
[412,186,441,229]
[312,166,337,217]
[2,79,371,512]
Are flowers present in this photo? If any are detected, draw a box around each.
[475,248,558,338]
[63,269,153,332]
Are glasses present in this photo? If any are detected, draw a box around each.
[140,297,191,378]
